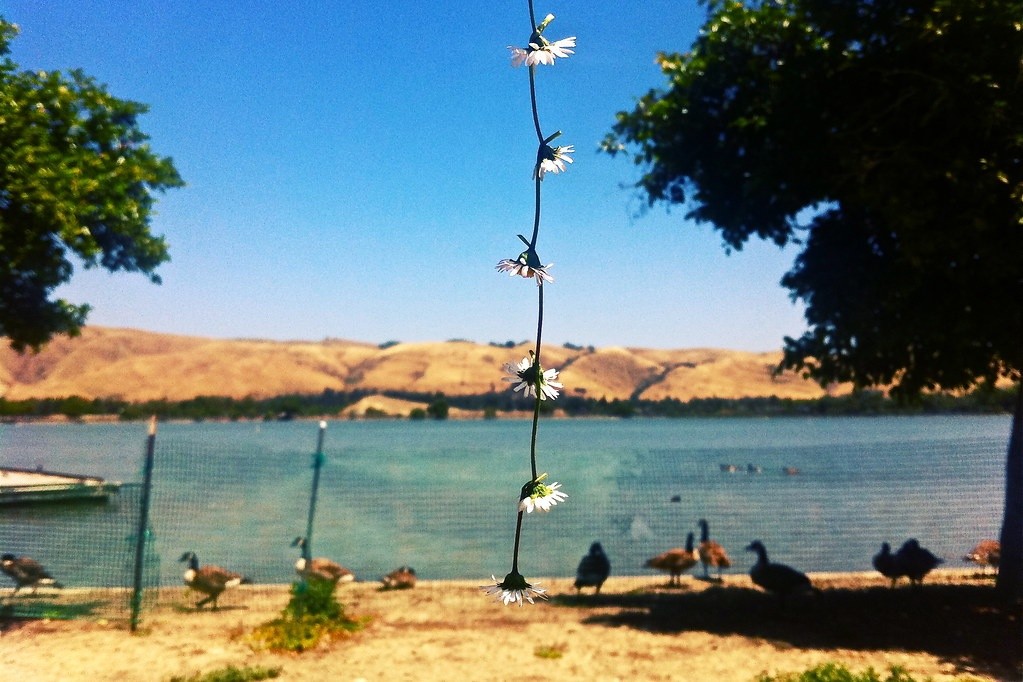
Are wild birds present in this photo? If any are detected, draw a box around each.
[176,551,255,612]
[375,566,418,591]
[871,537,946,594]
[780,463,802,476]
[0,552,64,602]
[740,538,830,612]
[288,535,367,594]
[638,518,736,589]
[962,538,1002,574]
[719,458,765,475]
[572,539,613,595]
